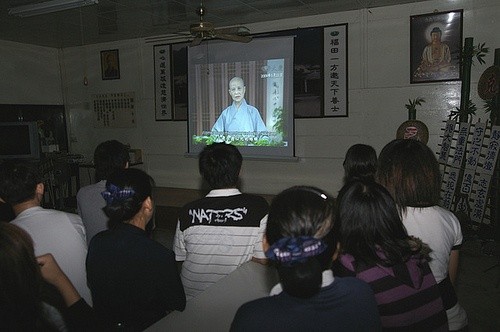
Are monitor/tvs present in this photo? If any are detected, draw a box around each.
[0,120,40,161]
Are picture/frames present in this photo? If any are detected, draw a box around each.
[100,48,121,80]
[409,8,464,84]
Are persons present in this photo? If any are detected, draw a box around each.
[229,185,381,332]
[329,138,469,332]
[0,139,186,332]
[173,141,271,301]
[211,77,267,131]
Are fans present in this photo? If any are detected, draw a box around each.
[156,1,254,46]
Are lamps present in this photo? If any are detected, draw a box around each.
[7,0,100,19]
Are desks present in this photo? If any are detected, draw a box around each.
[77,161,143,186]
